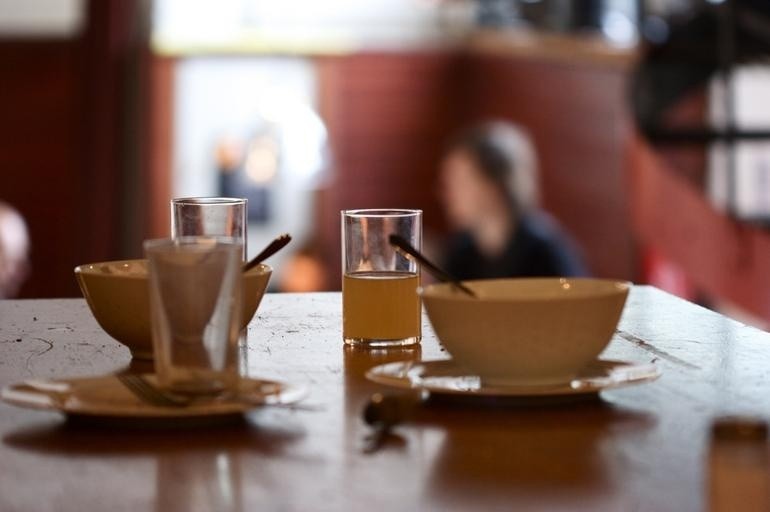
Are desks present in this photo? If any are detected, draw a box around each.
[0,282,770,512]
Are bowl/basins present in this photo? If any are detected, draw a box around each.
[74,259,273,362]
[419,277,629,388]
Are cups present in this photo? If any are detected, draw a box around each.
[170,196,248,260]
[143,239,244,406]
[340,208,422,347]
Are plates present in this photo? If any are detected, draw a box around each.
[366,355,665,408]
[1,375,308,429]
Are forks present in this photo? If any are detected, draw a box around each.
[114,371,326,427]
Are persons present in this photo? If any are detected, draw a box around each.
[436,118,587,284]
